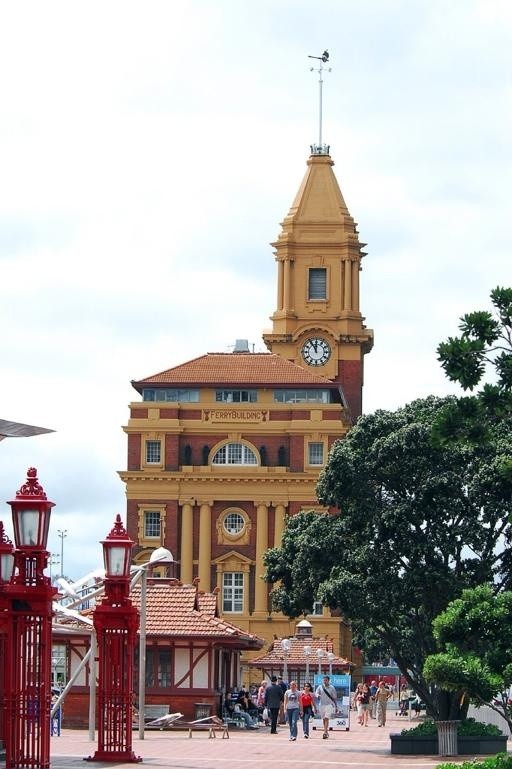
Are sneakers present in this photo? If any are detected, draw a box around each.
[358,717,385,728]
[248,724,259,730]
[289,737,296,741]
[257,722,266,726]
[304,734,308,739]
[323,733,329,739]
[271,731,278,734]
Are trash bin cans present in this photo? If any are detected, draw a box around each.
[433,720,462,757]
[193,703,214,724]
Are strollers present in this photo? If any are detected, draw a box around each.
[395,701,409,716]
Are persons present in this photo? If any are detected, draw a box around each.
[225,675,423,741]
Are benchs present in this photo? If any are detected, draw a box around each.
[227,711,259,729]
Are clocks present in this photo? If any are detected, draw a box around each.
[300,334,332,368]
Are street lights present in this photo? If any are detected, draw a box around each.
[0,469,59,768]
[328,652,334,675]
[317,648,324,679]
[48,528,67,577]
[87,515,146,751]
[281,639,291,685]
[57,578,96,741]
[303,646,311,685]
[140,547,175,740]
